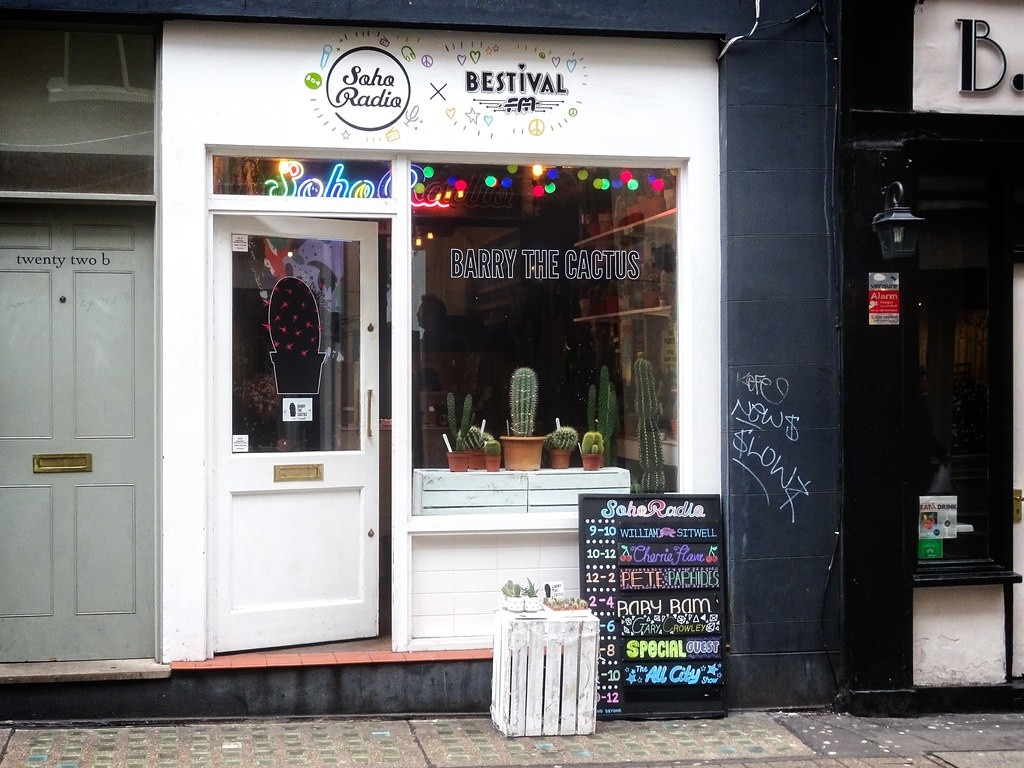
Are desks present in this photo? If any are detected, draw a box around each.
[615,436,678,466]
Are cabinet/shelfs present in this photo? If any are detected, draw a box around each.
[478,277,521,326]
[573,207,678,321]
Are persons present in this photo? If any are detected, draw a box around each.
[418,293,456,353]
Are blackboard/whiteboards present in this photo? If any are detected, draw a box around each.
[574,492,729,724]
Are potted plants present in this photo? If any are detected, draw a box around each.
[466,426,494,470]
[499,367,548,471]
[484,441,501,472]
[579,269,676,317]
[446,392,473,472]
[541,598,592,618]
[521,577,540,612]
[581,431,605,471]
[502,580,524,613]
[543,426,579,469]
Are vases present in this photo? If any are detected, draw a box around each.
[671,420,677,439]
[589,189,676,237]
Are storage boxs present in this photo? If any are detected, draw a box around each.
[490,608,600,737]
[413,467,631,516]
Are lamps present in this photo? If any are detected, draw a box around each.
[872,181,925,261]
